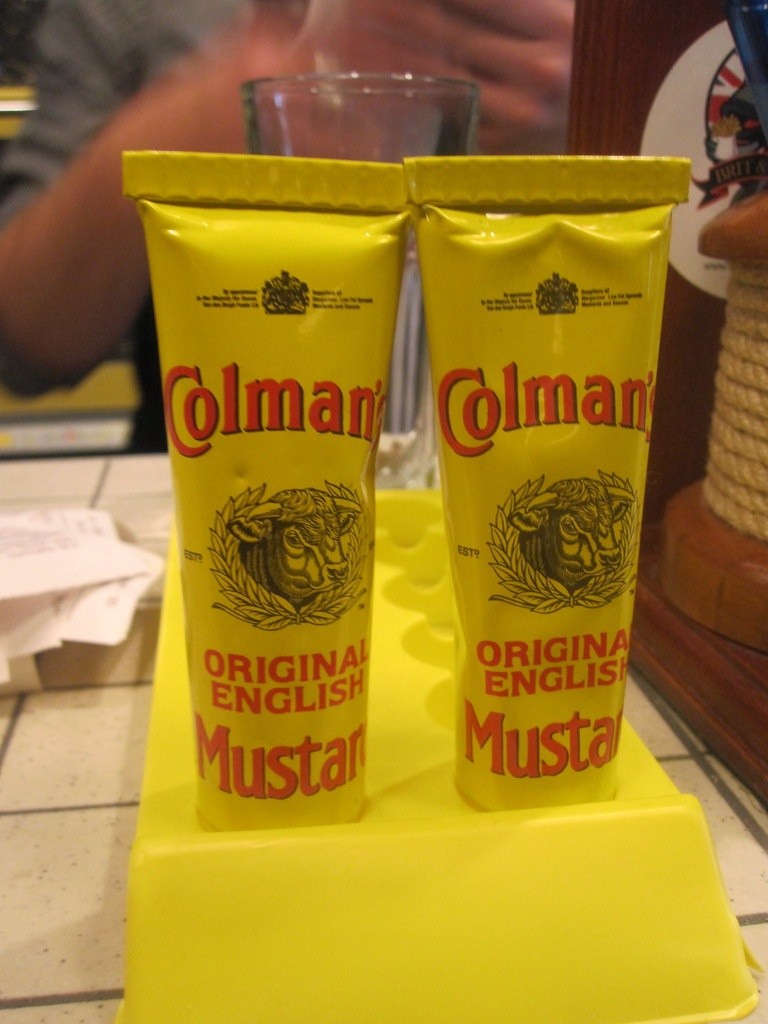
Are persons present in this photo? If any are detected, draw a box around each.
[0,0,576,456]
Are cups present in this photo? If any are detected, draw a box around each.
[239,67,483,490]
